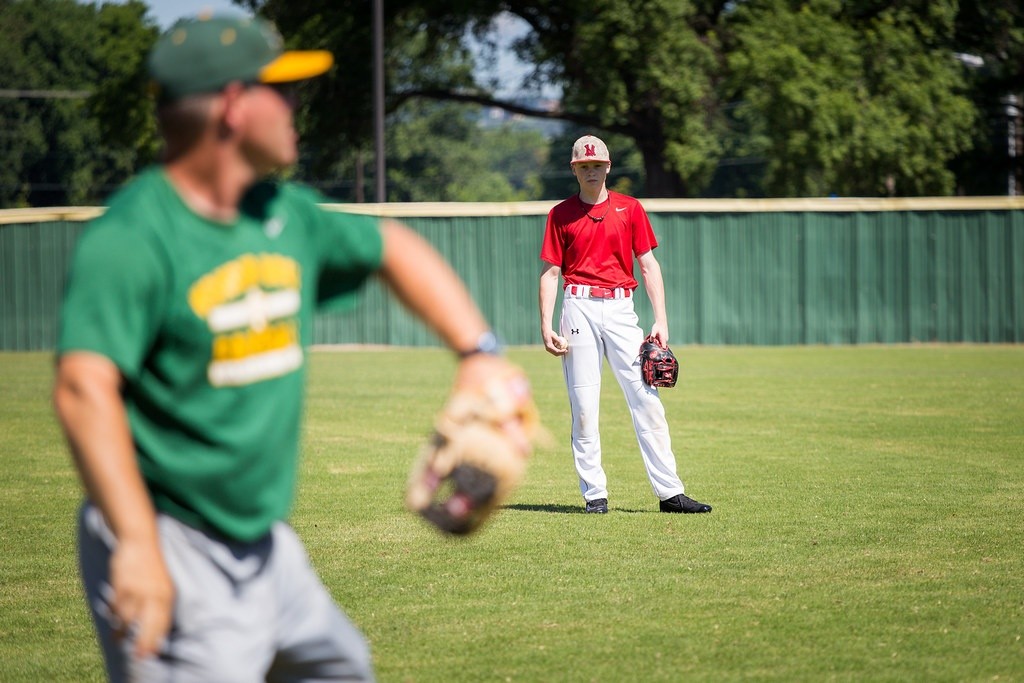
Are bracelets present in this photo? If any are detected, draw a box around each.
[456,330,505,360]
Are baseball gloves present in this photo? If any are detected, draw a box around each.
[638,333,679,388]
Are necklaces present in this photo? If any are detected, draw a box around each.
[577,188,611,221]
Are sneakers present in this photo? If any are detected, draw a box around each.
[660,494,712,513]
[586,498,608,513]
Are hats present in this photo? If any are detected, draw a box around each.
[147,8,333,105]
[571,135,609,164]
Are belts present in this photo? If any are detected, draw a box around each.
[571,286,630,299]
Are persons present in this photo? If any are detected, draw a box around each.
[537,134,714,515]
[50,15,543,683]
